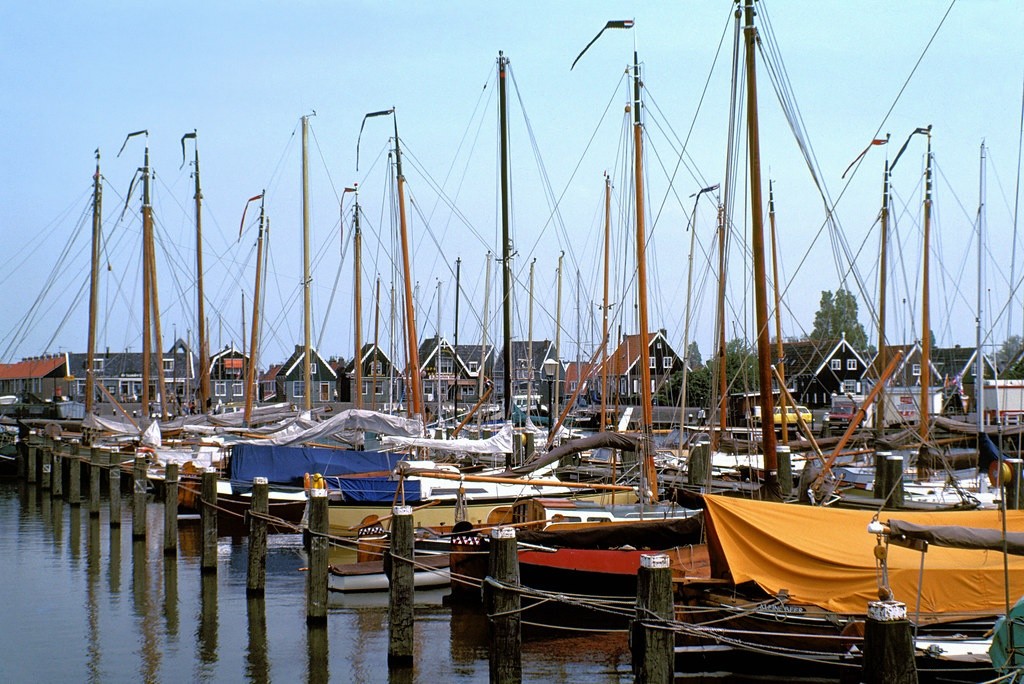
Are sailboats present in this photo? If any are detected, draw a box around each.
[2,3,1023,682]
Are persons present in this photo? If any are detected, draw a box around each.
[698,407,706,426]
[823,411,830,422]
[349,428,366,451]
[188,395,197,416]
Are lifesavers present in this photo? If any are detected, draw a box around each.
[312,473,323,489]
[136,447,158,465]
[303,472,310,491]
